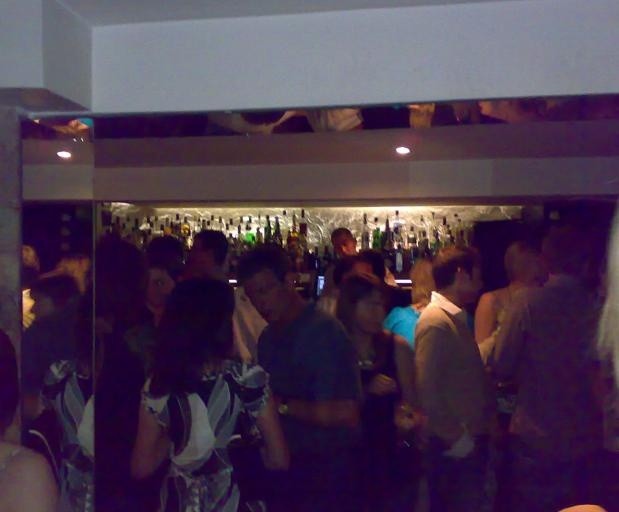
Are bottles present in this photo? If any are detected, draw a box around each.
[293,243,334,274]
[360,209,464,277]
[99,202,308,257]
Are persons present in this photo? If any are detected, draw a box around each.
[2,204,619,512]
